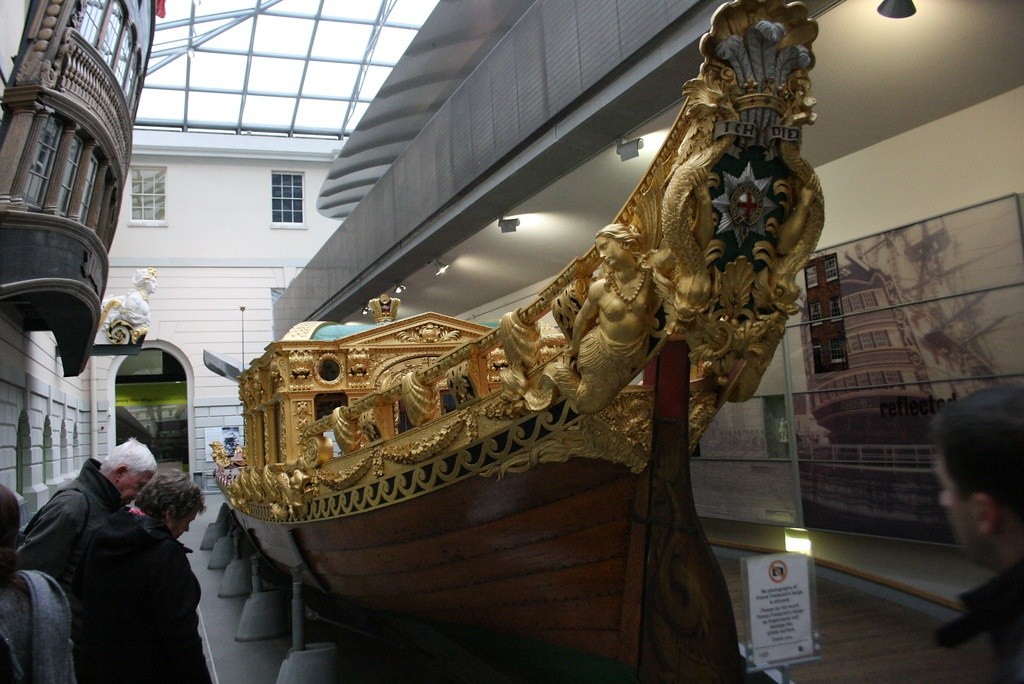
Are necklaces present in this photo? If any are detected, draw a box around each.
[610,272,646,313]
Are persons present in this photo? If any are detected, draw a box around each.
[70,468,212,684]
[14,438,158,599]
[932,386,1024,683]
[499,167,711,414]
[0,485,77,684]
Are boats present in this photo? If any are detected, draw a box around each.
[203,0,863,683]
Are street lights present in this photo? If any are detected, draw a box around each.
[239,306,245,371]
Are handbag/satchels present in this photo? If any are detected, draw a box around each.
[16,486,91,592]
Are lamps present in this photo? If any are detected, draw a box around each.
[498,216,520,233]
[878,0,916,19]
[616,136,644,162]
[432,259,450,276]
